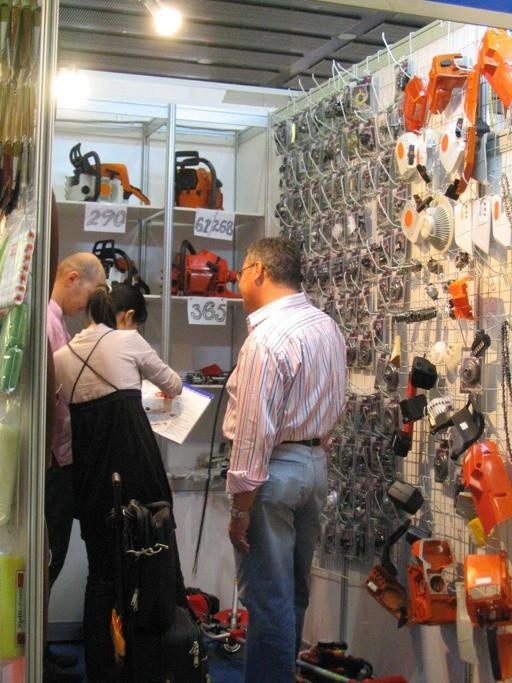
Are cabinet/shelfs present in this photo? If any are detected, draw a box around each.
[50,100,273,495]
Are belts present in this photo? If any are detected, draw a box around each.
[282,438,321,447]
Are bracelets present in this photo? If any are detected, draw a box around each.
[231,508,247,521]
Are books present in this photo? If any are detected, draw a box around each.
[138,378,213,445]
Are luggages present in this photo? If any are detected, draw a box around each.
[112,473,210,682]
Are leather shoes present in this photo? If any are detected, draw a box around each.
[43,647,78,667]
[42,663,85,682]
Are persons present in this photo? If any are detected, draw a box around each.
[46,251,106,682]
[52,282,185,683]
[221,237,347,683]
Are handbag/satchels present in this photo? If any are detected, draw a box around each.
[118,497,177,634]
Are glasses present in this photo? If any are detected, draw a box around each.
[235,262,265,282]
